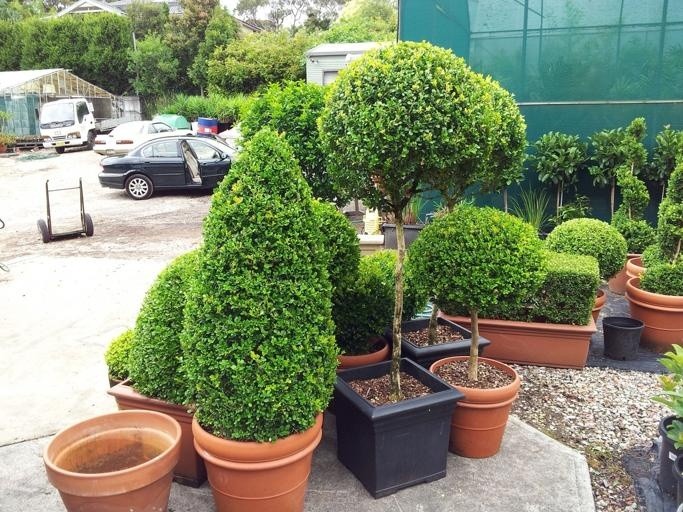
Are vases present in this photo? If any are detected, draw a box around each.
[41,409,182,511]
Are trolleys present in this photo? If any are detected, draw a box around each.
[37,177,93,243]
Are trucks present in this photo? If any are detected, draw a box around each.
[34,98,128,154]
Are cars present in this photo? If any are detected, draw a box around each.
[92,121,177,158]
[98,135,241,200]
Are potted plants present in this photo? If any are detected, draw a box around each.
[103,326,146,390]
[107,247,214,491]
[177,122,345,512]
